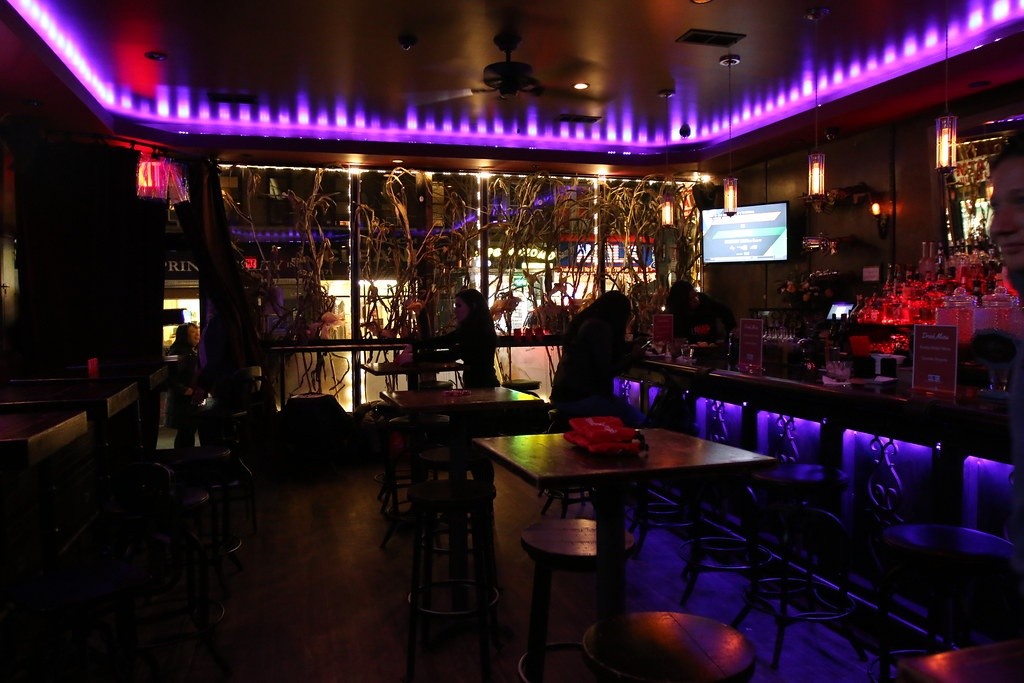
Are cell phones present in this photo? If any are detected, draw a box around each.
[641,340,653,348]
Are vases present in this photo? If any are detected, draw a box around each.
[785,292,805,306]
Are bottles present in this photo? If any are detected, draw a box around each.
[514,327,543,344]
[630,231,1024,380]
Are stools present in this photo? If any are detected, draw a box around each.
[378,414,1015,683]
[418,380,452,390]
[0,404,256,683]
[502,381,541,397]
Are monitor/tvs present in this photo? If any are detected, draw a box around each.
[700,201,792,265]
[826,303,856,321]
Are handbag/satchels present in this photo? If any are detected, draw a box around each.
[163,388,193,428]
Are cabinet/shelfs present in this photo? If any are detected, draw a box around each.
[796,183,873,290]
[729,306,827,380]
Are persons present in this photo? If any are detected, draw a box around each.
[548,291,657,428]
[166,323,200,449]
[991,126,1024,573]
[198,293,230,449]
[396,289,500,388]
[663,280,737,339]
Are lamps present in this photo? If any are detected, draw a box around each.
[657,88,676,230]
[718,55,742,217]
[802,8,828,203]
[935,0,957,179]
[869,199,890,240]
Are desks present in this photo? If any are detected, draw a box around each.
[270,334,564,411]
[359,359,777,683]
[0,353,188,683]
[893,638,1024,683]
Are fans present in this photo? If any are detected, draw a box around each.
[413,33,600,108]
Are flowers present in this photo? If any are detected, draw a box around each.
[773,266,816,305]
